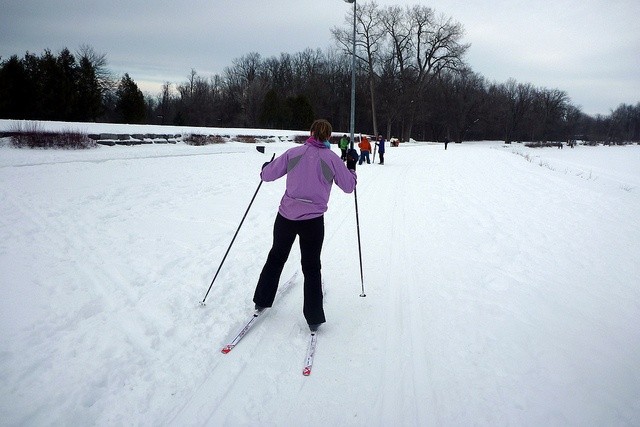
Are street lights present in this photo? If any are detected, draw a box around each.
[343,0,356,149]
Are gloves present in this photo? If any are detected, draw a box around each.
[347,148,359,170]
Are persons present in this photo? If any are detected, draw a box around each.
[375,135,385,164]
[339,135,349,161]
[444,136,448,149]
[359,136,371,164]
[253,119,359,332]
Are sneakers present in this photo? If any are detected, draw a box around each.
[307,319,322,331]
[254,301,270,310]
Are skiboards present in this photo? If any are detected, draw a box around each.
[222,274,316,375]
